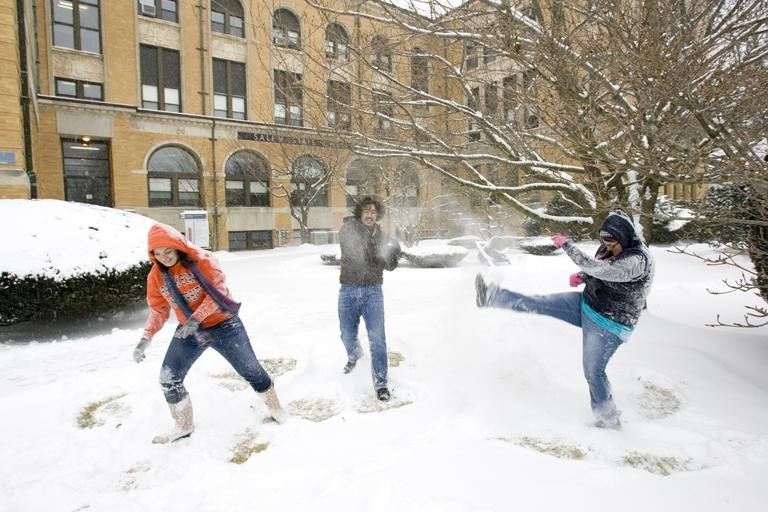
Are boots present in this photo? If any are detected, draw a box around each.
[152,395,194,443]
[257,382,288,424]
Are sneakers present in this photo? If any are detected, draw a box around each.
[475,274,487,307]
[344,362,356,374]
[590,416,620,430]
[376,387,391,402]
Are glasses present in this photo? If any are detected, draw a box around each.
[602,240,619,248]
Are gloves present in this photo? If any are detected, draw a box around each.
[134,337,150,363]
[174,316,200,340]
[570,272,582,287]
[552,233,567,247]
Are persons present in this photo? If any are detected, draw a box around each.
[475,209,654,431]
[132,223,288,444]
[338,195,401,403]
